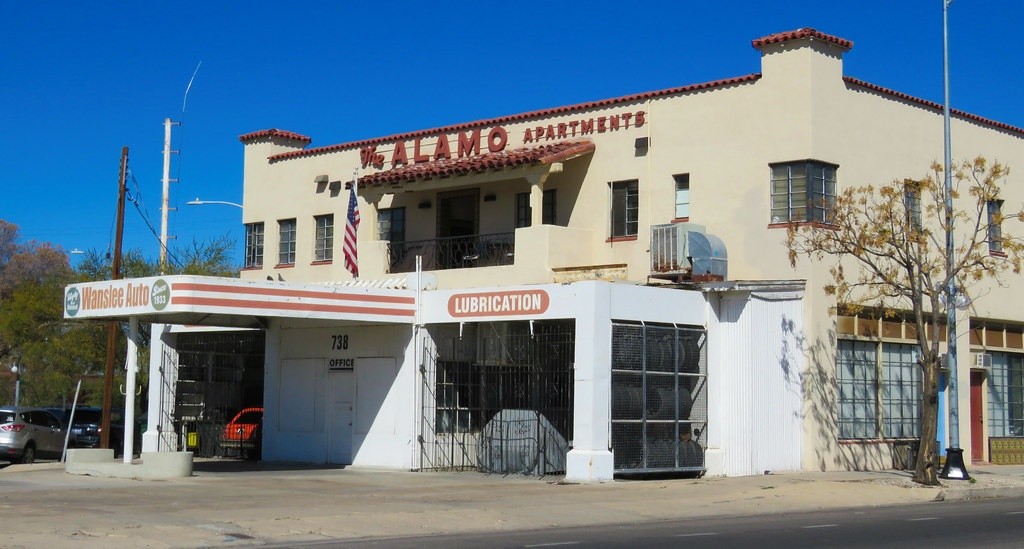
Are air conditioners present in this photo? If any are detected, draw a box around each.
[649,221,706,276]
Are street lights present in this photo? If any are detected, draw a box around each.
[11,361,22,405]
[935,279,972,479]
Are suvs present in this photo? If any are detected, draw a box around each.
[0,405,67,464]
[65,403,143,460]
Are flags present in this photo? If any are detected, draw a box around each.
[343,171,361,279]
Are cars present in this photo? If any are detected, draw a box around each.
[218,407,264,459]
